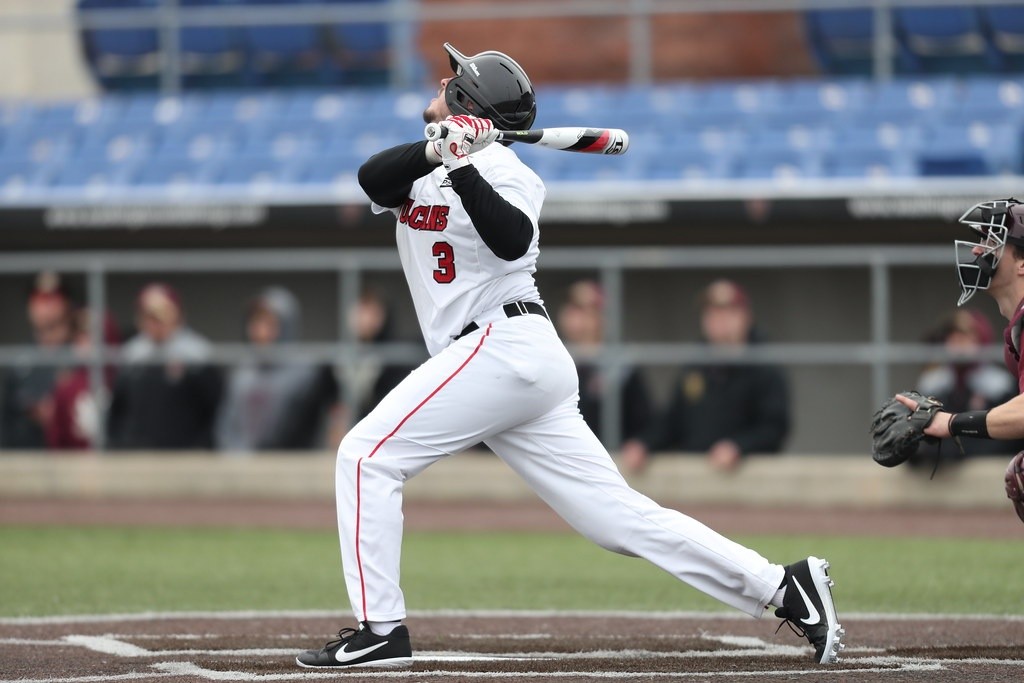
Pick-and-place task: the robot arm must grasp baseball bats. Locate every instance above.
[424,117,631,157]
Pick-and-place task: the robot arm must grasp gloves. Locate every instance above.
[433,116,499,164]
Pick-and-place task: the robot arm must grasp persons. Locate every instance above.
[874,198,1024,520]
[16,268,795,472]
[916,310,1020,411]
[296,41,846,669]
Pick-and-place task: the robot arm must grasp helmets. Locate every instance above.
[443,41,537,147]
[954,197,1024,307]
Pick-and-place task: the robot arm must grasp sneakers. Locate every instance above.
[774,555,846,666]
[296,623,413,668]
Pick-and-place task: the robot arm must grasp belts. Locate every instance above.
[451,303,549,340]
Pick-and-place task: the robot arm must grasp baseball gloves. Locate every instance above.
[867,389,968,480]
